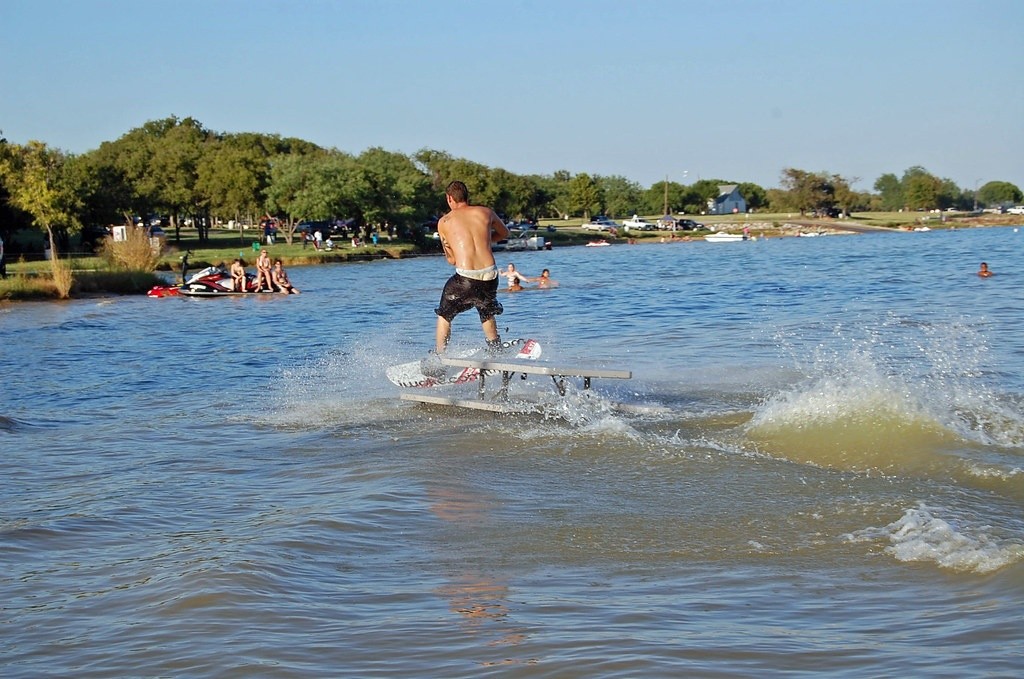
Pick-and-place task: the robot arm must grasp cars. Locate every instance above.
[581,219,621,231]
[590,216,608,221]
[505,218,540,231]
[671,218,704,232]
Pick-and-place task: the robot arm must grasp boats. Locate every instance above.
[704,230,751,242]
[178,268,296,299]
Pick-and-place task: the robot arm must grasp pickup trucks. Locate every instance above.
[623,214,655,231]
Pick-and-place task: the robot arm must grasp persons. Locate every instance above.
[240,204,1024,253]
[272,259,300,294]
[977,262,992,278]
[498,262,529,287]
[526,268,560,288]
[507,277,524,292]
[254,249,274,293]
[230,259,248,293]
[433,180,510,360]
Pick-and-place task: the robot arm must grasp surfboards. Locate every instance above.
[386,338,542,389]
